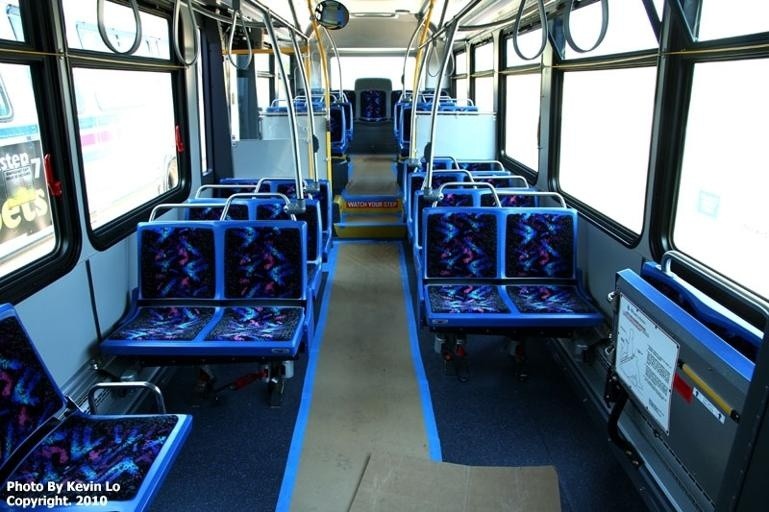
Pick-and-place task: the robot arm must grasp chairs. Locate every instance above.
[89,88,357,413]
[390,88,610,384]
[0,301,195,512]
[355,89,392,129]
